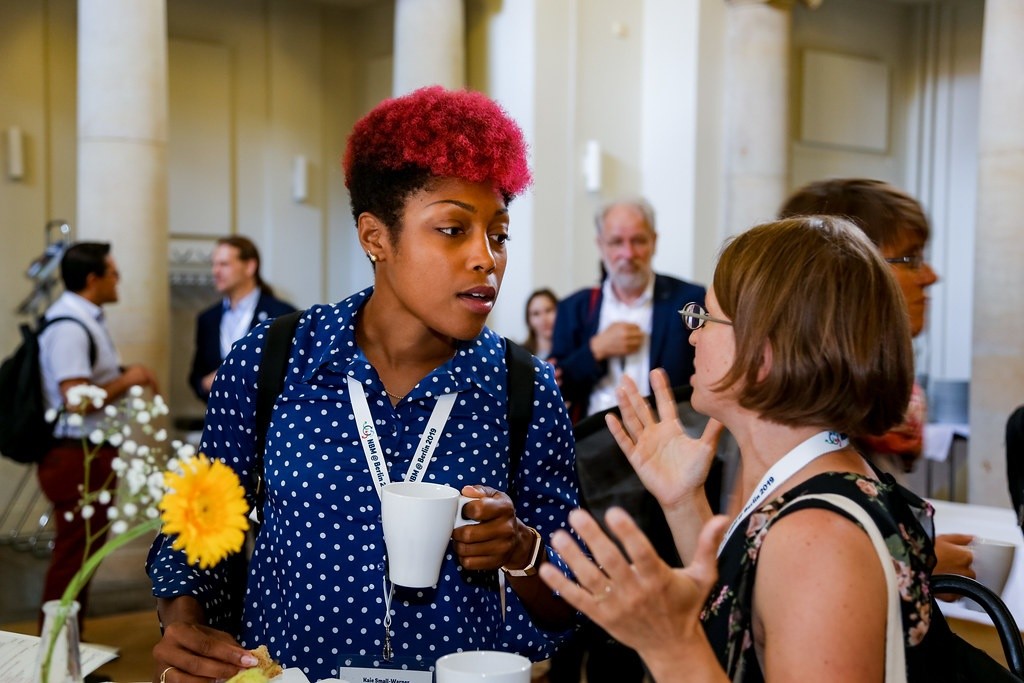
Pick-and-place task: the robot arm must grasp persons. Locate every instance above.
[39,243,159,640]
[521,289,558,363]
[538,216,939,683]
[550,197,710,424]
[145,86,584,683]
[776,178,976,602]
[188,237,295,402]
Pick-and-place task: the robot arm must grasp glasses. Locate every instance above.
[678,302,734,329]
[887,254,922,270]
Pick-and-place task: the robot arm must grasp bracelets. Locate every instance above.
[501,526,545,577]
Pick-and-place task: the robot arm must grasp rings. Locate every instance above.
[160,666,175,683]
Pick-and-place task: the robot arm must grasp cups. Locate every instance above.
[380,482,482,587]
[963,537,1015,613]
[435,650,532,682]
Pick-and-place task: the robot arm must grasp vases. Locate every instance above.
[29,601,81,683]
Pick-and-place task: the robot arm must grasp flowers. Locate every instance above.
[39,383,249,683]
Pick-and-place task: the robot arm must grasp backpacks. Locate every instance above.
[0,315,97,462]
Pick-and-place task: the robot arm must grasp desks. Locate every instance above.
[921,418,972,503]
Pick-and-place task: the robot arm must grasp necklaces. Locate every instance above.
[385,389,404,400]
